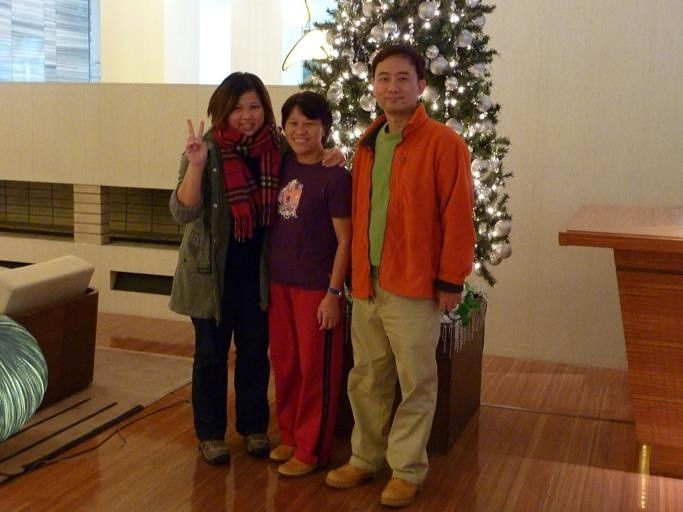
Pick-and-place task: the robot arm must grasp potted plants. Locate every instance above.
[269,1,511,460]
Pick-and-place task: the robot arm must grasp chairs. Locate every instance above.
[0,255,99,412]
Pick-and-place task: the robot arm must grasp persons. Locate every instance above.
[166,71,346,466]
[262,88,350,479]
[322,42,478,511]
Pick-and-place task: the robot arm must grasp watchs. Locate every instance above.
[325,287,343,298]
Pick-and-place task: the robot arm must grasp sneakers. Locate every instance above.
[199,439,230,464]
[270,445,296,460]
[278,458,317,476]
[247,433,270,457]
[380,478,421,507]
[325,462,386,489]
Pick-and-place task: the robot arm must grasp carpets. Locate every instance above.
[1,345,193,491]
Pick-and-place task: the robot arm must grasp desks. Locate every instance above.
[560,203,683,447]
